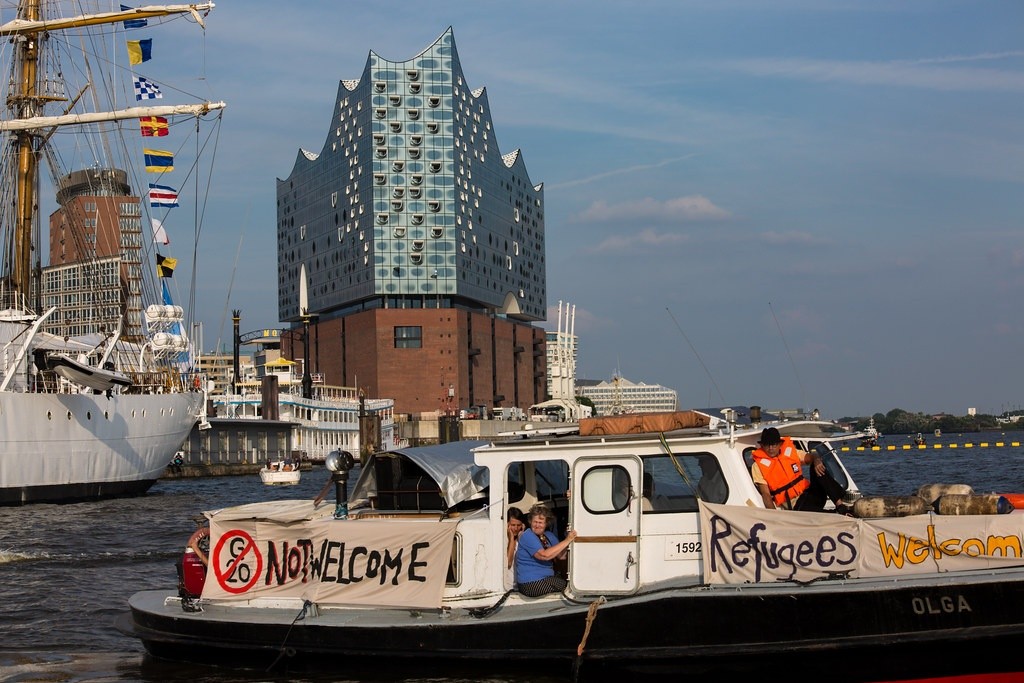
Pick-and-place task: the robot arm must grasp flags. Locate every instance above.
[133,76,162,101]
[140,115,169,136]
[151,220,169,246]
[156,254,177,279]
[127,38,153,67]
[144,148,174,174]
[120,6,148,30]
[149,183,179,208]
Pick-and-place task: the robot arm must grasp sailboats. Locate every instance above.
[0,0,226,506]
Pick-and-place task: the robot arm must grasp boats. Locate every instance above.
[258,461,302,488]
[127,408,1023,683]
[915,432,926,445]
[861,436,877,448]
[934,428,941,438]
[859,417,878,439]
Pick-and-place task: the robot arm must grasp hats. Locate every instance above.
[757,427,784,444]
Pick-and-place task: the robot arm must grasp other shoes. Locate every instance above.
[835,500,857,512]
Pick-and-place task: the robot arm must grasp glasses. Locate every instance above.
[763,441,779,448]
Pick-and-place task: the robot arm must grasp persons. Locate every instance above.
[693,453,727,505]
[813,409,820,421]
[751,427,860,518]
[515,505,576,597]
[643,471,654,512]
[507,506,525,590]
[174,452,184,465]
